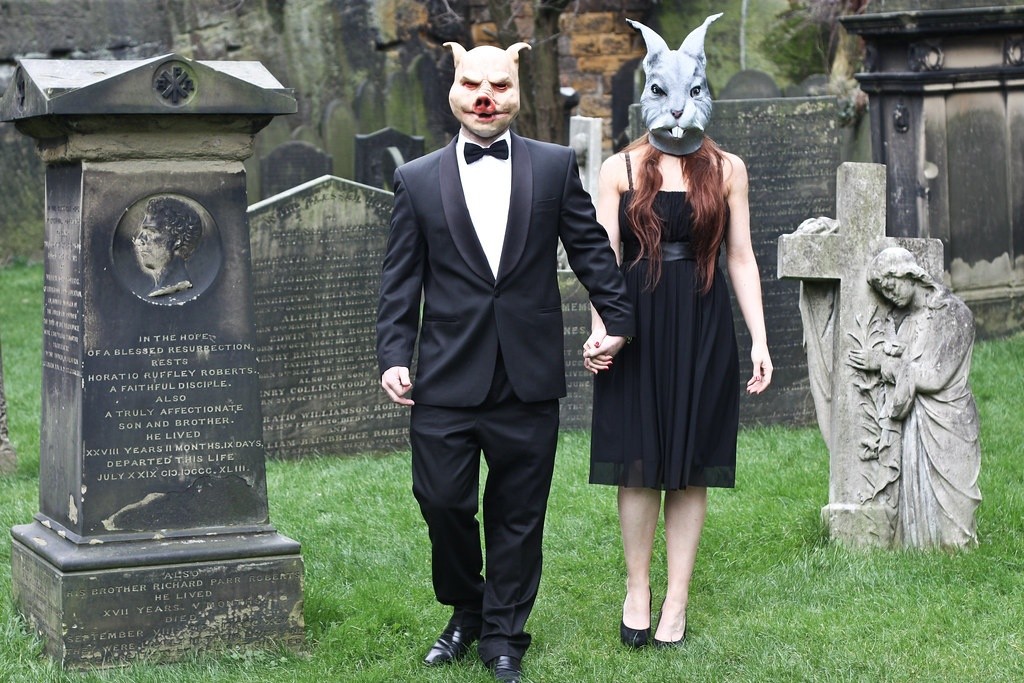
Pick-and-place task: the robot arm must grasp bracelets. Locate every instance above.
[625,336,632,344]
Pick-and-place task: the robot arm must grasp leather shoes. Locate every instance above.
[424,622,482,668]
[493,655,520,683]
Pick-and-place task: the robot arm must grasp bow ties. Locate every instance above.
[464,139,509,165]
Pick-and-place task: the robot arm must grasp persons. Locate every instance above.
[376,40,636,683]
[849,247,983,553]
[582,12,773,653]
[135,197,202,297]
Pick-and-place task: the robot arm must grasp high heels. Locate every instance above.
[653,596,687,653]
[621,585,652,649]
